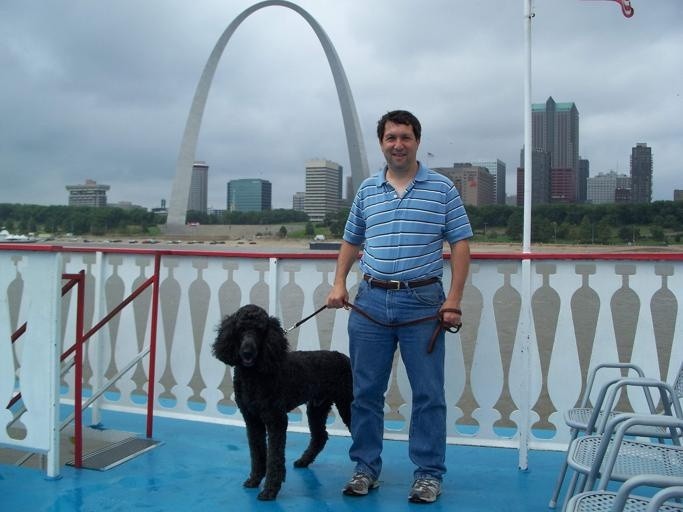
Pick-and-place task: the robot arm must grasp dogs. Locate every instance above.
[210,303,354,500]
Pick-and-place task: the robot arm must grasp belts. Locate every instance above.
[358,270,444,291]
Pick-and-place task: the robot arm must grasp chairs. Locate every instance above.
[549,358,683,512]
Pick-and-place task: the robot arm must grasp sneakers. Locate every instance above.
[407,476,443,506]
[339,472,382,497]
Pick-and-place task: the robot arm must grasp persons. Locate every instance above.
[323,108,474,504]
